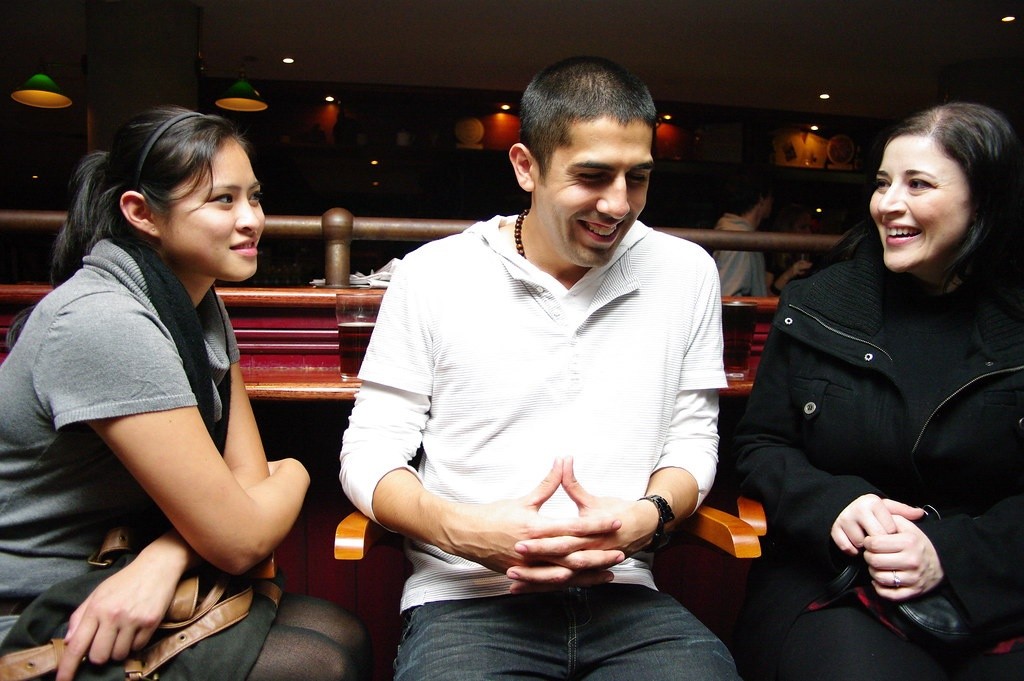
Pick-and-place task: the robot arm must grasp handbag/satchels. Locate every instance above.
[801,497,1024,655]
[0,525,284,681]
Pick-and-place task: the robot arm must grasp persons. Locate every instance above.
[0,104,370,681]
[731,102,1024,681]
[712,178,812,303]
[339,56,741,681]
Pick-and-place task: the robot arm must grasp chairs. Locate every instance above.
[335,512,760,563]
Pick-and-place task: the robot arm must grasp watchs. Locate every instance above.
[636,496,678,553]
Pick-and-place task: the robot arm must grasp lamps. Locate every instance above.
[200,61,270,113]
[10,53,86,109]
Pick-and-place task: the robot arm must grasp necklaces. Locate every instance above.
[514,207,534,262]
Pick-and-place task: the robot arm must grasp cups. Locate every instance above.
[720,300,759,381]
[334,295,382,379]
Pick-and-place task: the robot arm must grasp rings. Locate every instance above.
[889,571,909,593]
